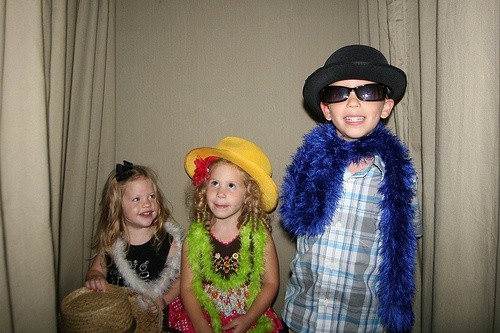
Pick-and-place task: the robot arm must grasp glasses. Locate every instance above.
[319,84,388,103]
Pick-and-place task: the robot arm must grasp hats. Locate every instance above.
[184,136,278,214]
[60,283,161,333]
[303,45,407,124]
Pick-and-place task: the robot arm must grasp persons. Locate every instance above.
[167,136,284,333]
[82,160,182,333]
[277,45,419,333]
